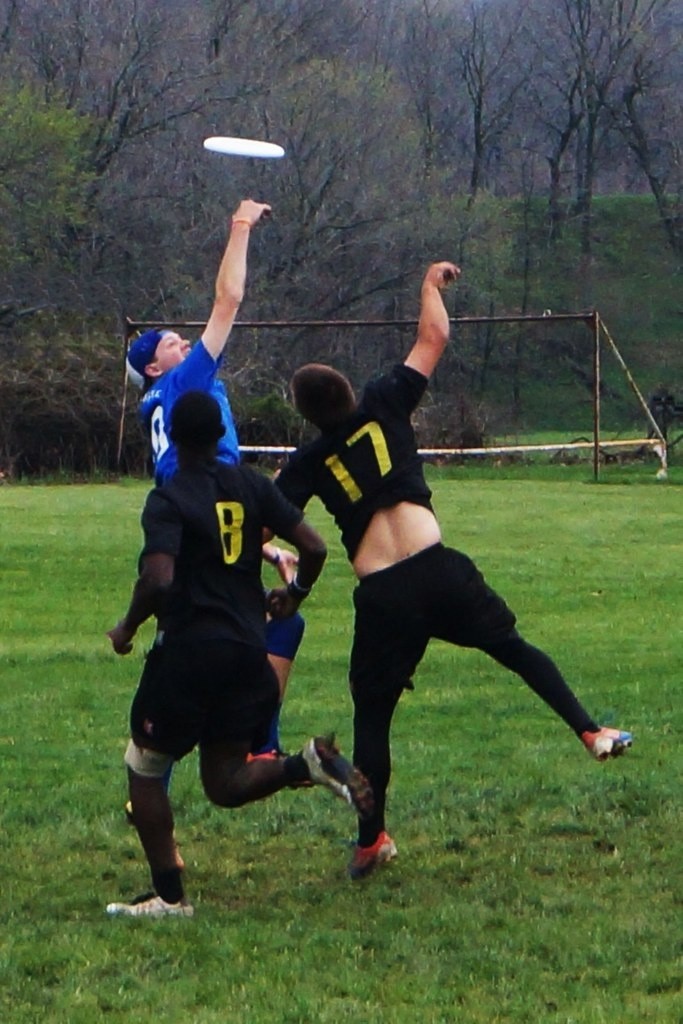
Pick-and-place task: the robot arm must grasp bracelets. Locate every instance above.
[232,218,253,231]
[270,546,280,566]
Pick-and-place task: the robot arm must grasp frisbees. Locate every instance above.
[203,136,286,159]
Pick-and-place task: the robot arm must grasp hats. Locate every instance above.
[129,327,170,395]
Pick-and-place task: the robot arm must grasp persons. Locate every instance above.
[127,198,306,816]
[263,261,634,877]
[107,392,376,919]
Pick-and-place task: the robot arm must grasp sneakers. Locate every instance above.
[302,737,375,820]
[346,832,399,879]
[584,724,633,760]
[106,896,197,915]
